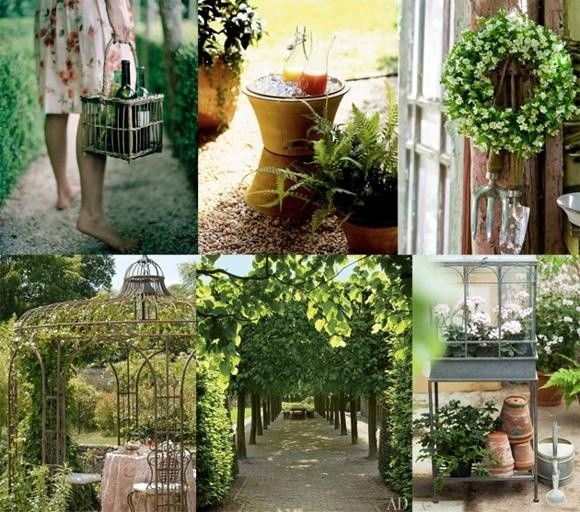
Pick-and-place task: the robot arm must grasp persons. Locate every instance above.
[33,1,143,254]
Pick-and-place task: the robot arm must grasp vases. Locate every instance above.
[531,370,566,406]
[482,395,535,477]
[240,71,352,157]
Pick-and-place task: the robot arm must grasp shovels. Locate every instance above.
[499,73,532,255]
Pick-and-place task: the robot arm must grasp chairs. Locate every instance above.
[126,441,193,512]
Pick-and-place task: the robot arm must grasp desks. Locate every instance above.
[106,449,191,512]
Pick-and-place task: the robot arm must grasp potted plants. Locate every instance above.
[411,398,503,493]
[239,77,400,254]
[199,0,272,133]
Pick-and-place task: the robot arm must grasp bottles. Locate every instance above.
[284,22,336,96]
[106,60,150,151]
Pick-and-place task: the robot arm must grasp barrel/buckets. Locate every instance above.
[538,421,575,487]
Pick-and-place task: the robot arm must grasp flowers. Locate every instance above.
[431,255,580,372]
[438,8,580,162]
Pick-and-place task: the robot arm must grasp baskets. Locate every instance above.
[81,37,166,166]
